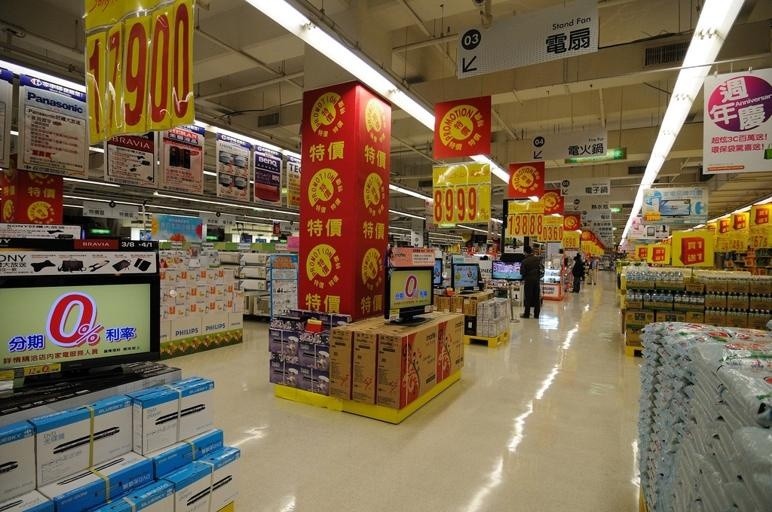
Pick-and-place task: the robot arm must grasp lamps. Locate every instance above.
[618,0,745,250]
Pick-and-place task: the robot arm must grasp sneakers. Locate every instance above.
[520,312,541,319]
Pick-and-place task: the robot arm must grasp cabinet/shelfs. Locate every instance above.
[540,256,566,301]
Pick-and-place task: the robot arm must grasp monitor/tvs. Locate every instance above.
[451,263,480,293]
[434,258,442,288]
[492,260,524,281]
[385,267,433,324]
[0,238,160,393]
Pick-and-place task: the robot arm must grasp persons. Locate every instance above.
[519,246,543,319]
[587,256,598,286]
[569,256,584,294]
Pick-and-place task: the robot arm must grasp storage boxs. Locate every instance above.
[158,248,246,320]
[3,375,242,512]
[328,311,465,409]
[435,288,490,336]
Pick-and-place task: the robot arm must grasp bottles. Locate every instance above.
[619,269,771,318]
